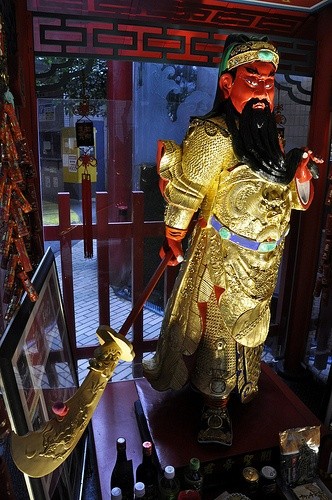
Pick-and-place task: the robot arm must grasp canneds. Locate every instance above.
[241,467,261,493]
[260,466,277,494]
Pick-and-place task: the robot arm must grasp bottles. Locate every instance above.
[136,441,156,491]
[110,438,134,500]
[134,482,148,500]
[159,465,180,500]
[184,458,203,493]
[111,487,122,500]
